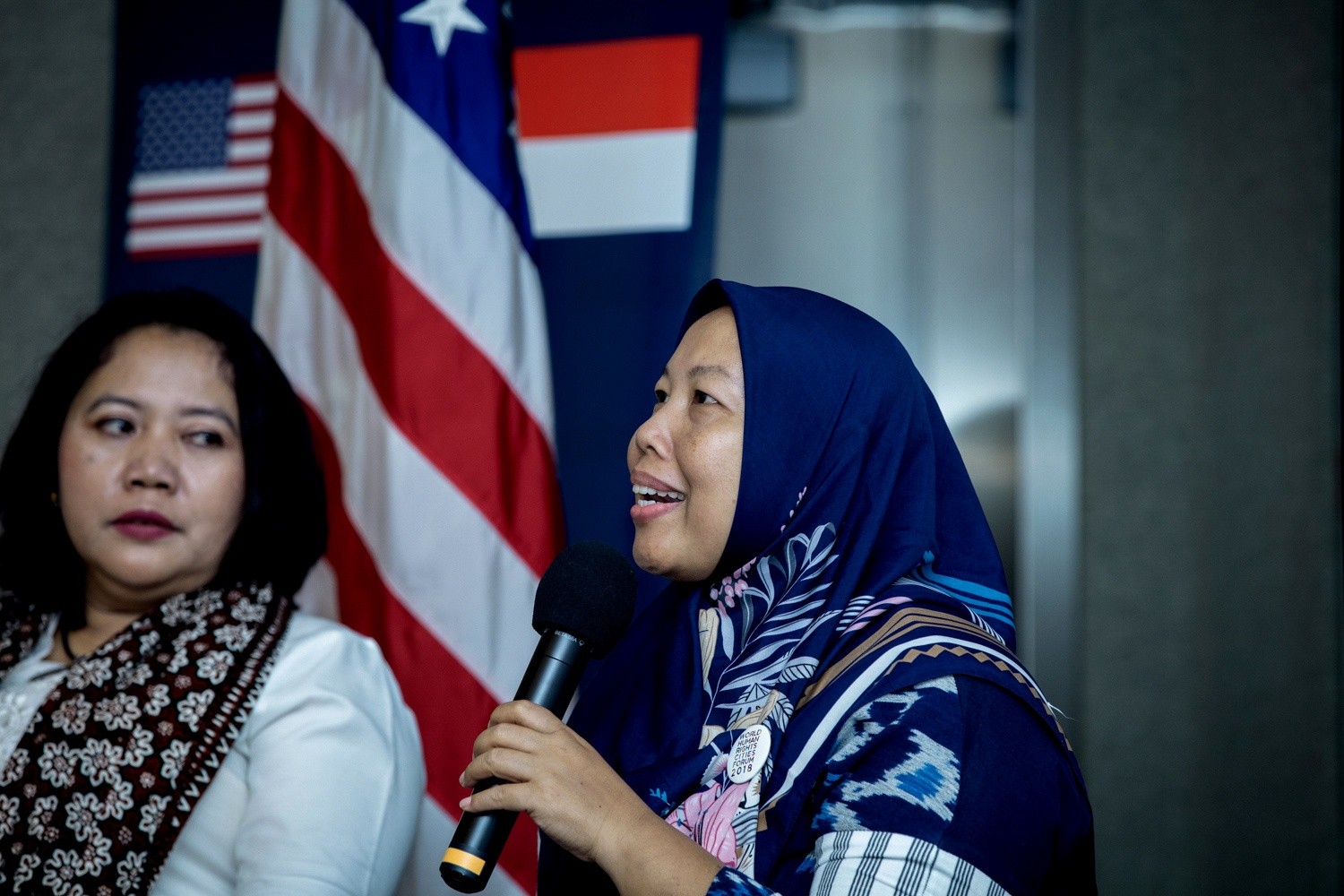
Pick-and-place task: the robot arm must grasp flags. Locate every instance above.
[251,1,564,896]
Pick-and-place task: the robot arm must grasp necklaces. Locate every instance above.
[58,630,81,661]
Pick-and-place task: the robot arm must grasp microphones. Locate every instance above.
[439,539,638,893]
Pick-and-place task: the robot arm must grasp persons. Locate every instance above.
[457,276,1096,896]
[0,288,429,896]
